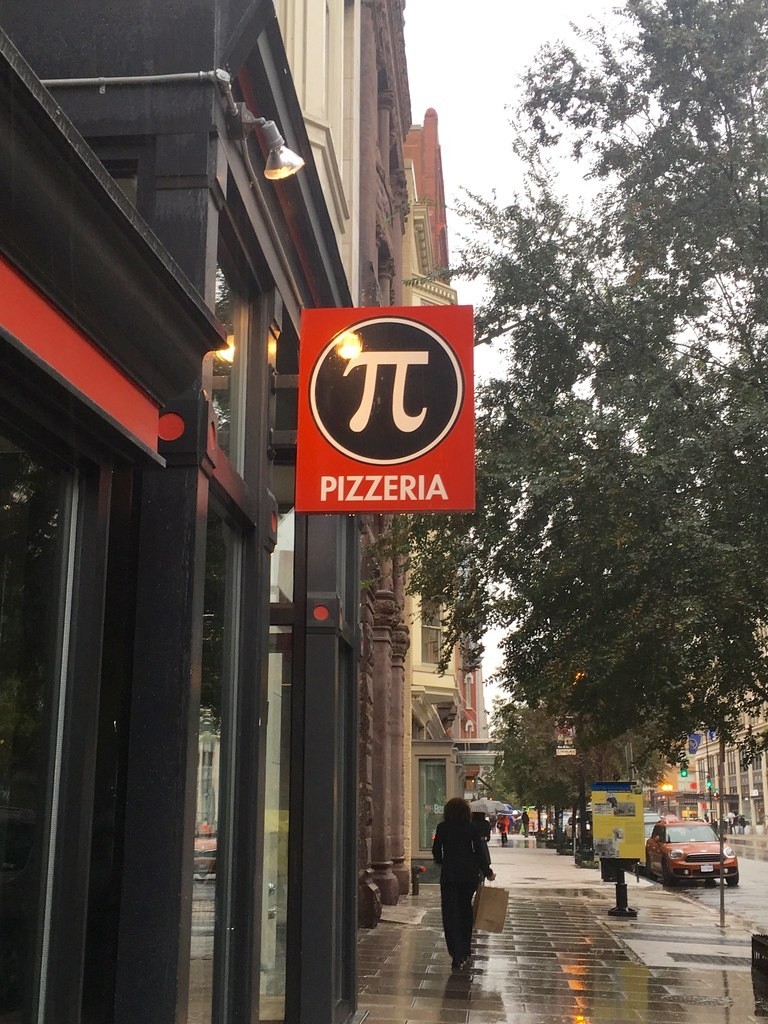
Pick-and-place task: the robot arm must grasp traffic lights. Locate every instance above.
[707,776,711,787]
[680,763,689,777]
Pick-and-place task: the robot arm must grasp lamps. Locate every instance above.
[226,101,306,181]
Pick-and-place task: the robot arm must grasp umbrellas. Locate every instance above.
[466,799,522,815]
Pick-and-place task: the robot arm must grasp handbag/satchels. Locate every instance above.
[472,879,509,933]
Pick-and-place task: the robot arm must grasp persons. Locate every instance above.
[432,797,497,967]
[470,800,493,865]
[497,808,529,848]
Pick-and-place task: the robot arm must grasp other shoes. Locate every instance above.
[452,950,472,968]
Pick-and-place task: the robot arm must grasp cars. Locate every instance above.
[521,805,595,848]
[643,811,661,868]
[644,816,739,888]
